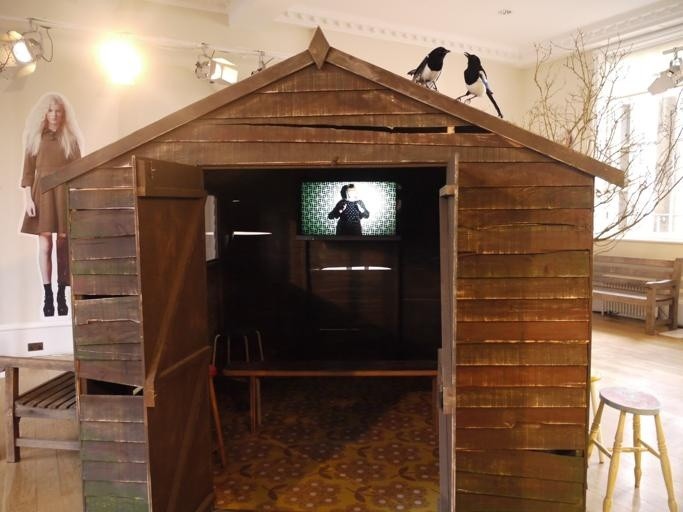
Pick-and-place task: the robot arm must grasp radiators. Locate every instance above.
[602,281,647,321]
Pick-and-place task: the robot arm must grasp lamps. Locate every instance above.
[193,44,239,86]
[1,18,53,78]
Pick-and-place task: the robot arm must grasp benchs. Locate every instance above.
[225,360,438,435]
[1,356,84,464]
[594,255,682,334]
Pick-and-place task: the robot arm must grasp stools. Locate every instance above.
[587,375,680,512]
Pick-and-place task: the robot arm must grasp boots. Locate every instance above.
[43,283,54,317]
[56,284,68,316]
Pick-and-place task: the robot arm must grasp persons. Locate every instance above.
[328,184,369,235]
[20,92,81,317]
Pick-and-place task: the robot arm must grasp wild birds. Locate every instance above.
[407,46,451,92]
[455,52,503,118]
